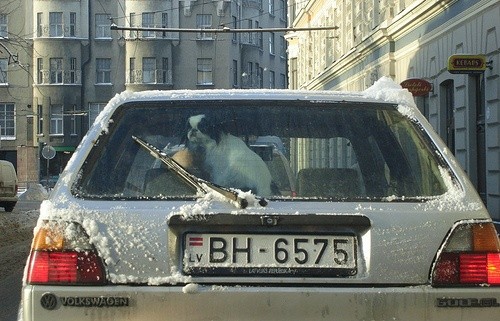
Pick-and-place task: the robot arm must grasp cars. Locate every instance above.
[18,88,499,321]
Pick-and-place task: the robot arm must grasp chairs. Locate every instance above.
[144,166,199,196]
[296,166,362,198]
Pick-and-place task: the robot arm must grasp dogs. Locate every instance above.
[178,114,282,197]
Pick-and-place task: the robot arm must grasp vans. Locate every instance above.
[0,160,18,212]
